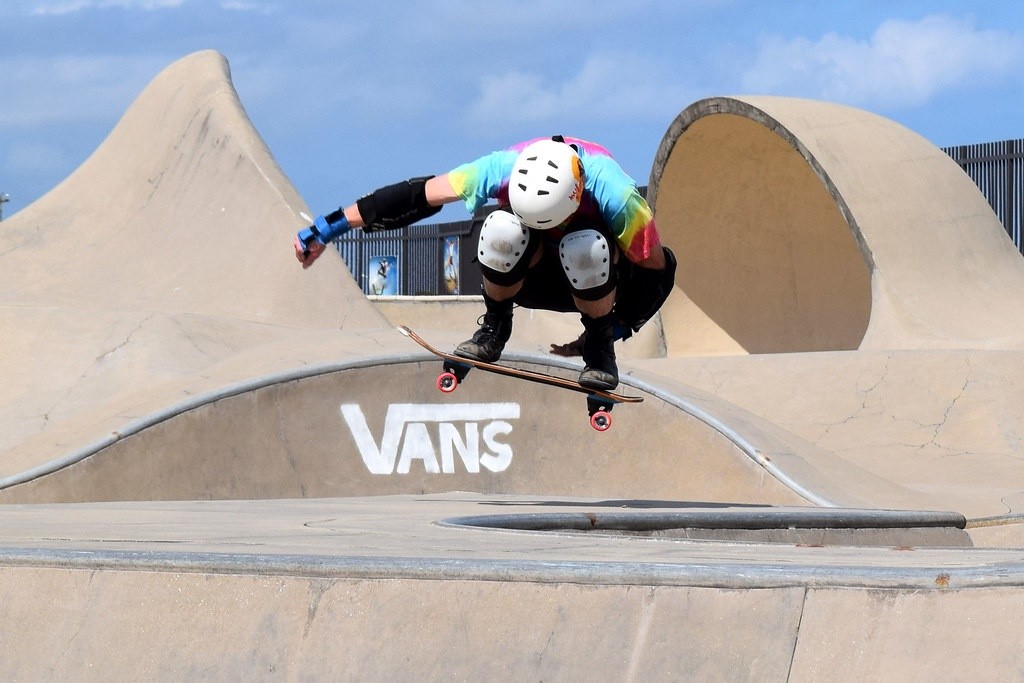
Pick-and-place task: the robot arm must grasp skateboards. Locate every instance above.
[398,321,644,431]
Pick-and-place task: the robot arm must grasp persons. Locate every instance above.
[444,237,458,284]
[294,135,677,389]
[378,258,388,274]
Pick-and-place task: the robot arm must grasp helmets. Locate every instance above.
[507,134,586,232]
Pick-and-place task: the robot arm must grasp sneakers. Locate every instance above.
[579,348,618,390]
[454,319,513,363]
